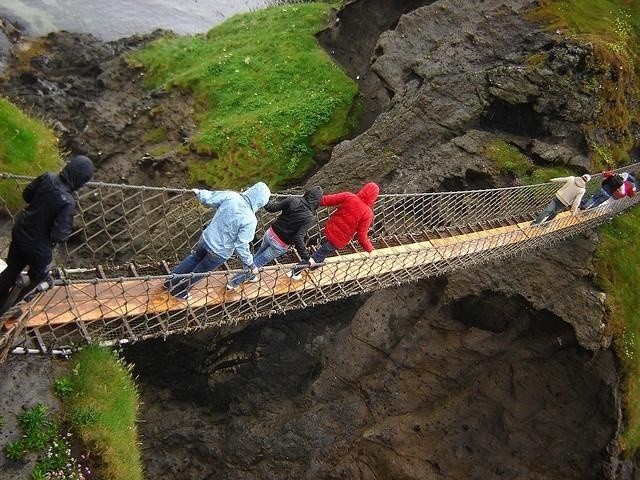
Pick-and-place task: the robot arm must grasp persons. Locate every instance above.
[162,182,271,296]
[580,171,628,211]
[286,182,380,281]
[529,174,591,229]
[0,156,94,317]
[226,186,324,290]
[600,171,640,208]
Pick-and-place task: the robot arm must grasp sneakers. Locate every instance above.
[285,270,302,279]
[244,275,260,283]
[225,284,244,292]
[162,283,192,301]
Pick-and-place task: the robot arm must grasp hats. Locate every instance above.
[582,174,591,182]
